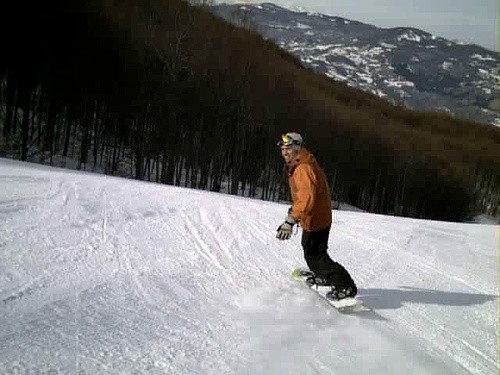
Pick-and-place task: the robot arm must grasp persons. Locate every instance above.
[276,132,357,301]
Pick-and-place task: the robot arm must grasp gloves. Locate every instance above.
[276,217,295,240]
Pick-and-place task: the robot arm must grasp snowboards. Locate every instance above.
[292,267,372,314]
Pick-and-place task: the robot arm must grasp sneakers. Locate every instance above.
[325,281,357,300]
[306,274,334,286]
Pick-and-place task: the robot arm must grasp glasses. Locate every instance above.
[276,135,303,146]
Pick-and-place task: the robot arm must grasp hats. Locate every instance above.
[286,133,304,149]
